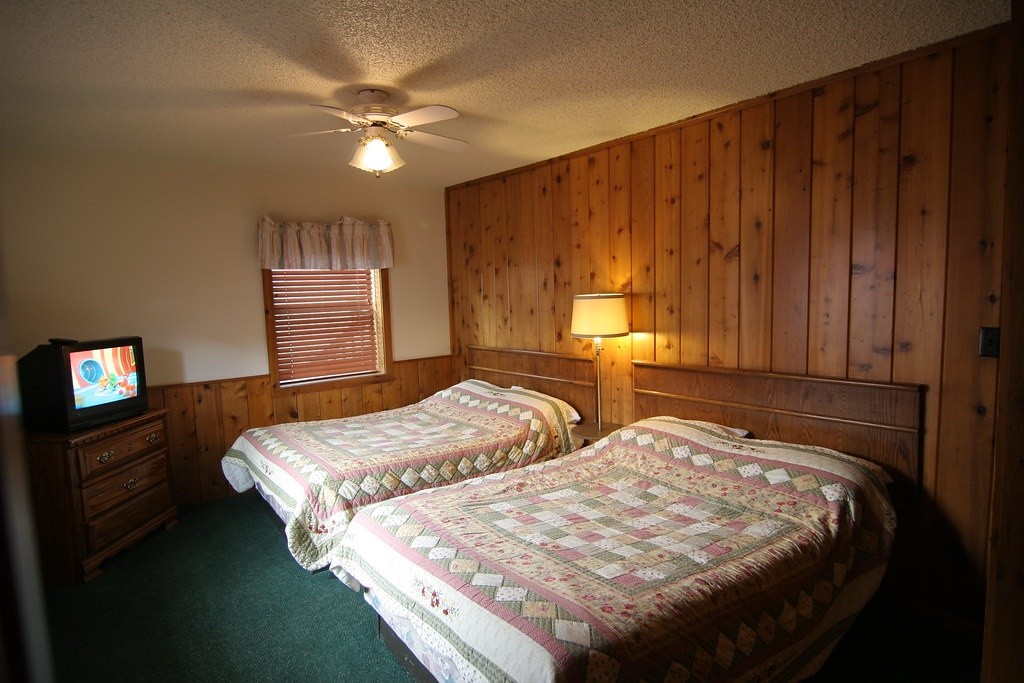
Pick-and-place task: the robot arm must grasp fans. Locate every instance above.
[256,89,468,153]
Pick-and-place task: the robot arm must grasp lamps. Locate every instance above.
[347,135,407,179]
[570,292,630,432]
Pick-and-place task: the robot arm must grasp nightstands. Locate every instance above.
[570,422,626,447]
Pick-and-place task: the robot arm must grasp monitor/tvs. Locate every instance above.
[17,335,149,437]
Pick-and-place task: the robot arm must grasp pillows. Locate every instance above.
[609,416,880,520]
[444,378,581,430]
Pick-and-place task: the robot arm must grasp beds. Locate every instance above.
[220,346,598,573]
[328,359,929,683]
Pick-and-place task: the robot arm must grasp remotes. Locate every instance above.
[47,338,78,345]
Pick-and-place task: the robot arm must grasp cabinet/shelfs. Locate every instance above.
[22,407,181,585]
